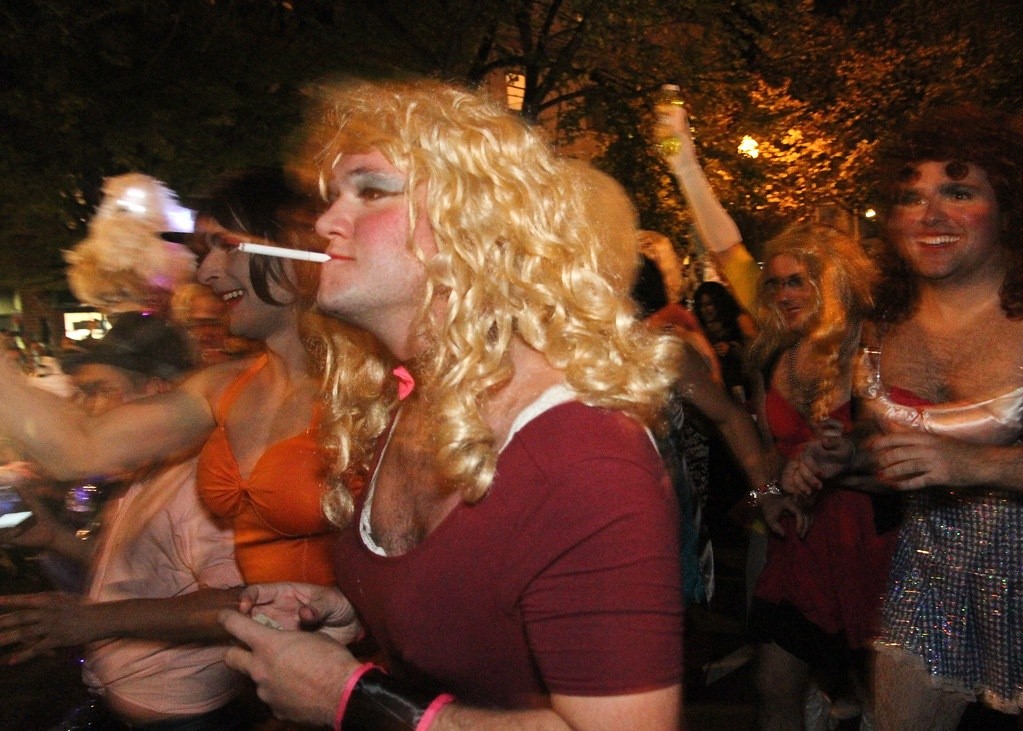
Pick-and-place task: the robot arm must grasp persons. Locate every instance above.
[0,78,1023,730]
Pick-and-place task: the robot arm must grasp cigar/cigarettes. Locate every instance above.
[238,243,331,263]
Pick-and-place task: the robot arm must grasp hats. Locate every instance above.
[59,310,194,385]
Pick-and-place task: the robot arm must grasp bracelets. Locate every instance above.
[747,481,781,508]
[334,663,454,731]
